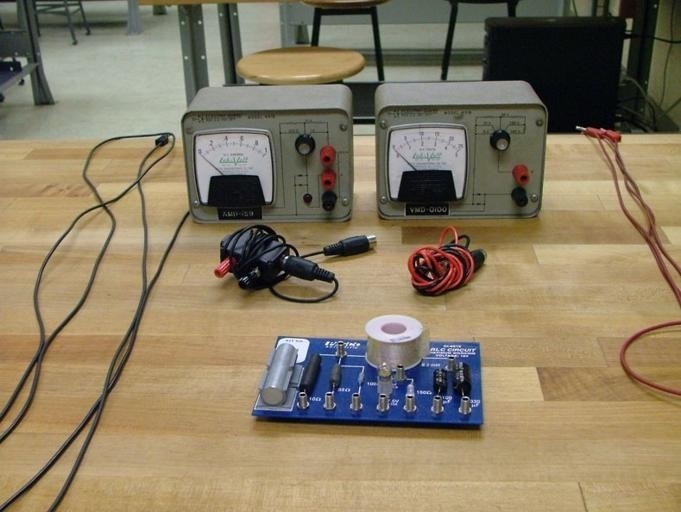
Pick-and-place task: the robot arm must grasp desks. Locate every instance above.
[0,134,681,512]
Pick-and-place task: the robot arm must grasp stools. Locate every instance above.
[440,1,521,79]
[311,4,390,81]
[235,46,367,85]
